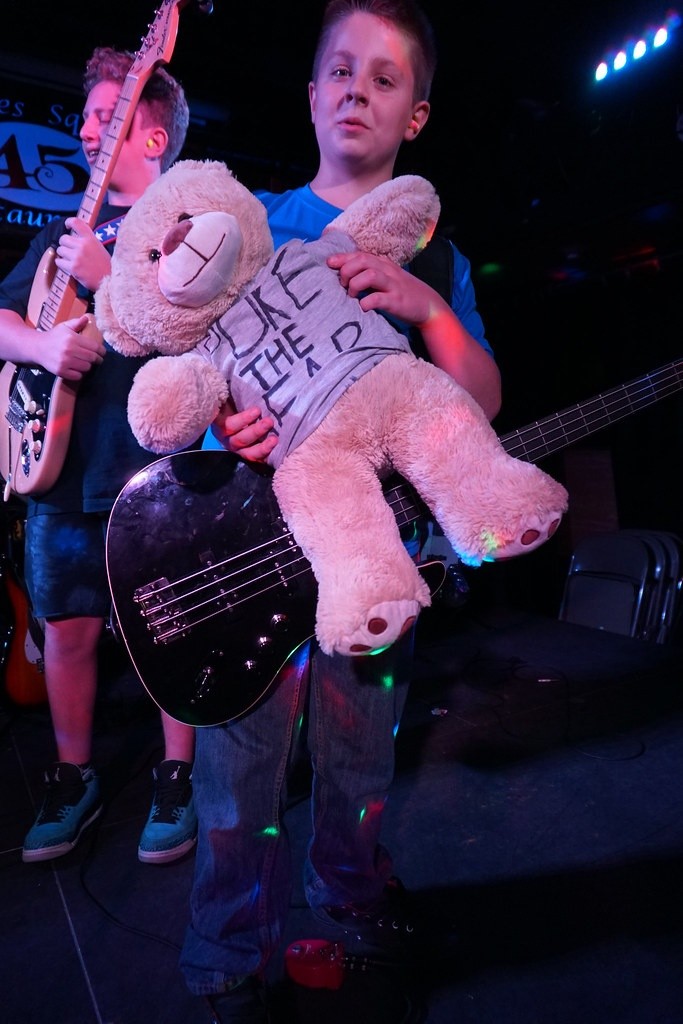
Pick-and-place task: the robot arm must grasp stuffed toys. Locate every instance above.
[92,158,569,661]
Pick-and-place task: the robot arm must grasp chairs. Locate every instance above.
[558,528,683,645]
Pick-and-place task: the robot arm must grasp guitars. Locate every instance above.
[97,358,683,729]
[0,1,185,500]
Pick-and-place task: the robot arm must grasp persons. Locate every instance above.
[0,50,220,863]
[183,11,503,1024]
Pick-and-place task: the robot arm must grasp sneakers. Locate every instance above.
[134,759,197,864]
[199,972,277,1024]
[307,876,475,980]
[21,758,106,863]
